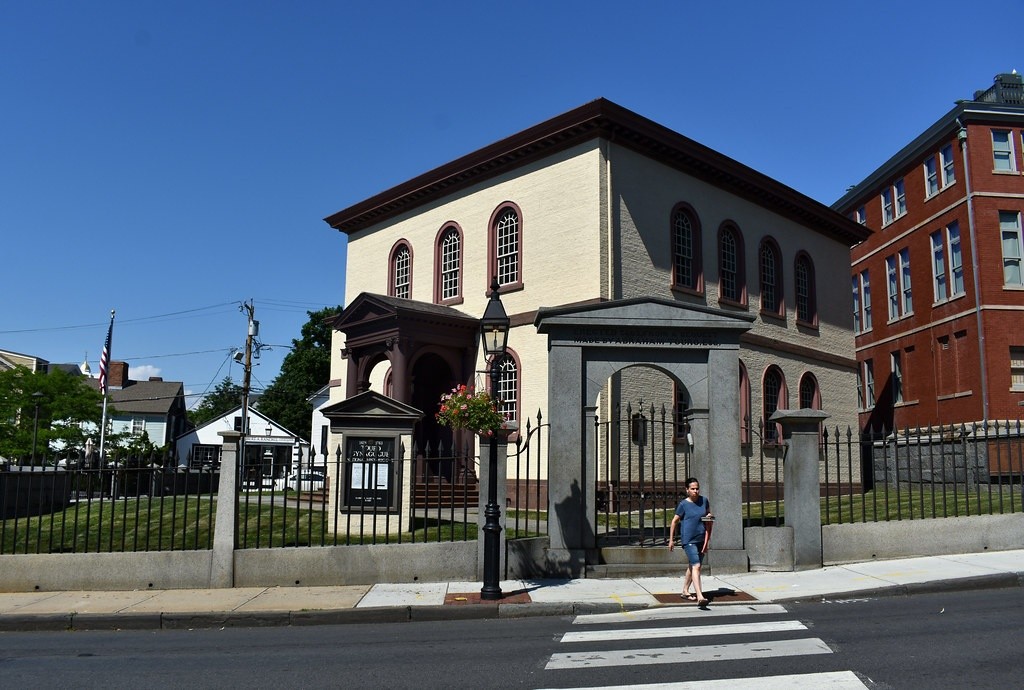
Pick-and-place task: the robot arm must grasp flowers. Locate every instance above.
[435,384,511,437]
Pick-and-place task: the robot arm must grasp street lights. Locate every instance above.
[479,276,511,600]
[30,388,43,471]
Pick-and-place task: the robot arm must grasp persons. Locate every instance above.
[669,478,713,606]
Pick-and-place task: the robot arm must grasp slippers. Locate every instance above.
[680,594,695,601]
[698,599,710,606]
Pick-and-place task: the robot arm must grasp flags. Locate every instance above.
[100,324,112,396]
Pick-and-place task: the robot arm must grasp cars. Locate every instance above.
[274,470,325,492]
